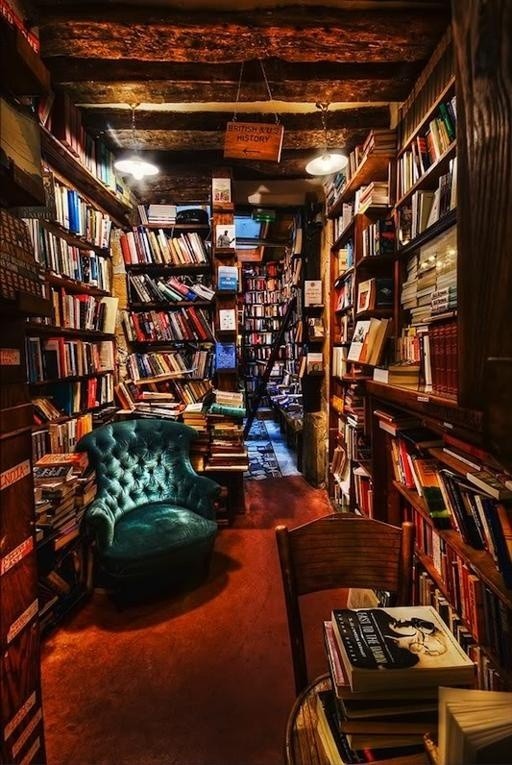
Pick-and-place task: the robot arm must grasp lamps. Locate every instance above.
[113,154,159,182]
[304,151,350,177]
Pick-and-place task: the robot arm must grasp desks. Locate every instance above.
[285,660,375,765]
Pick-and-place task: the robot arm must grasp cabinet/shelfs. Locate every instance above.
[319,149,398,522]
[130,220,215,399]
[365,119,509,432]
[21,114,134,632]
[366,398,512,643]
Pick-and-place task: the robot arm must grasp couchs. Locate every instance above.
[77,416,222,611]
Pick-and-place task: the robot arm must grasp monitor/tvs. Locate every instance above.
[234,216,262,250]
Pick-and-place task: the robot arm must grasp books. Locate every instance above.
[114,379,213,472]
[123,306,215,343]
[127,350,207,380]
[333,202,353,243]
[395,324,459,401]
[304,280,323,308]
[374,403,512,601]
[332,311,352,378]
[241,249,304,425]
[396,495,512,678]
[136,202,178,224]
[323,127,397,211]
[220,309,236,331]
[21,218,115,293]
[19,333,120,384]
[391,95,456,196]
[315,605,475,764]
[34,281,119,335]
[307,353,323,375]
[418,564,510,692]
[216,342,235,369]
[127,271,215,303]
[218,266,237,290]
[210,390,250,471]
[435,686,512,765]
[362,219,396,256]
[347,319,389,367]
[329,385,371,516]
[36,82,131,207]
[397,158,458,243]
[42,170,116,252]
[32,414,101,542]
[398,223,459,320]
[356,277,377,315]
[119,225,209,265]
[31,373,116,423]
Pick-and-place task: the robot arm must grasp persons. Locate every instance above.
[218,226,234,247]
[309,361,322,376]
[331,605,449,672]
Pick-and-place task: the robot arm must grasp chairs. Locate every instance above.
[275,509,419,703]
